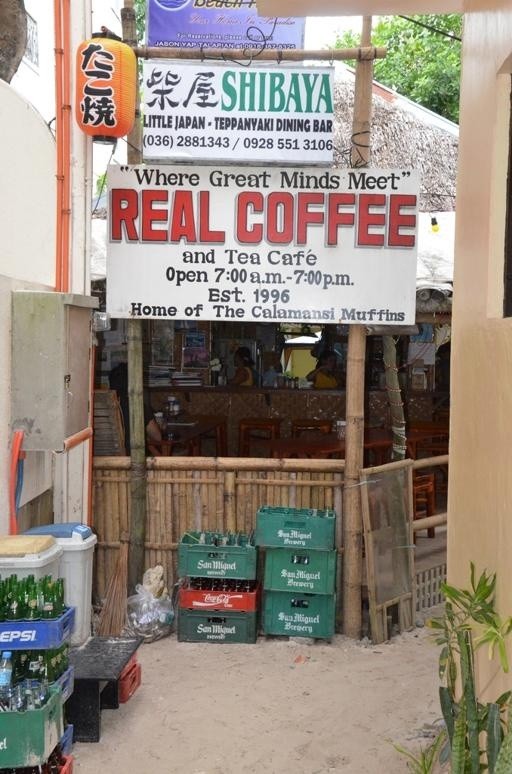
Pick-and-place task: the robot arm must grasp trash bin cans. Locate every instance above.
[0,523,97,647]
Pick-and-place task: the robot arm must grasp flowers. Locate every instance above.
[209,357,222,371]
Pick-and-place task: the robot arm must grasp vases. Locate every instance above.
[211,370,218,385]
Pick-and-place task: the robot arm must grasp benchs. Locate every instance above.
[64,633,145,742]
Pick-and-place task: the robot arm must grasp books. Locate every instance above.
[144,362,203,387]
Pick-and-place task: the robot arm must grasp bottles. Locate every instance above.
[192,530,257,594]
[323,506,331,517]
[0,575,70,710]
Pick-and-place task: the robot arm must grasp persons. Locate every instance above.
[368,358,382,386]
[108,363,162,457]
[307,351,338,388]
[230,346,255,386]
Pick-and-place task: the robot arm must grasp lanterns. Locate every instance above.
[73,31,138,146]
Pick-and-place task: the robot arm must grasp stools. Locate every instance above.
[237,417,332,457]
[384,459,435,543]
